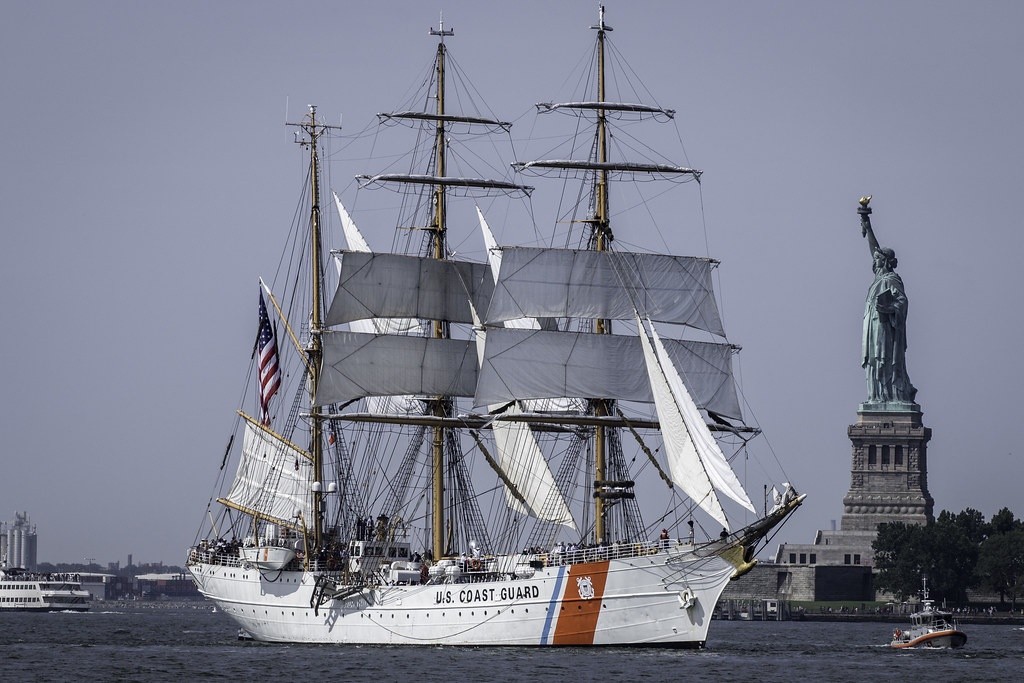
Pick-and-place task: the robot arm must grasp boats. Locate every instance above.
[890,575,968,650]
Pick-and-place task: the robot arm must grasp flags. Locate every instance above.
[257,285,282,428]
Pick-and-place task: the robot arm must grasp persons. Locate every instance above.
[860,215,918,403]
[792,599,1019,618]
[198,512,731,587]
[895,627,902,641]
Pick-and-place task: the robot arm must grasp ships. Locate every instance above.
[0,511,92,611]
[187,0,807,652]
